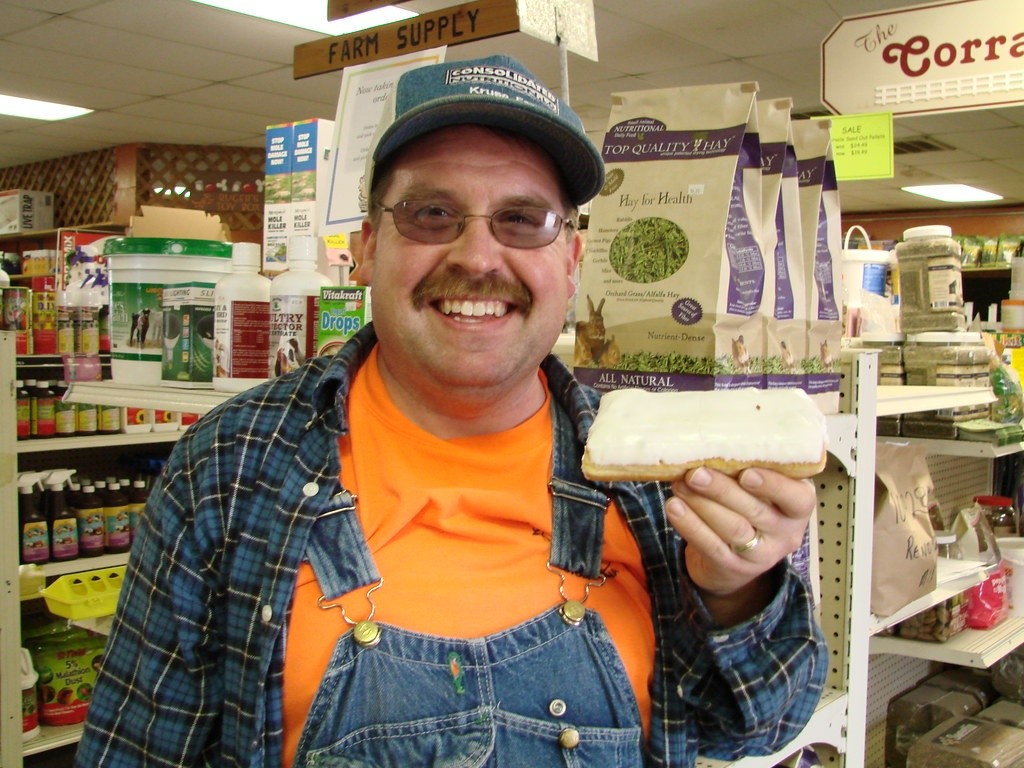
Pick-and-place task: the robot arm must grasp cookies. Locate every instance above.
[581,388,827,483]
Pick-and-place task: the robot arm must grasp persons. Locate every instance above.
[72,54,829,768]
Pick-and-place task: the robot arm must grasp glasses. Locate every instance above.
[373,197,574,249]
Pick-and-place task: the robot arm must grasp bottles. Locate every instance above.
[974,494,1020,539]
[20,641,41,744]
[899,529,969,643]
[15,379,173,566]
[212,241,273,393]
[903,331,991,440]
[268,235,333,380]
[860,331,905,437]
[895,226,966,334]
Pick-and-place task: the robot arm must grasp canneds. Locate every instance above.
[0,275,110,358]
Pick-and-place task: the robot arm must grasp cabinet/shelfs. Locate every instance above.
[0,330,1024,768]
[0,222,129,279]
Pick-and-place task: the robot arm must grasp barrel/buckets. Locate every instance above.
[838,224,891,314]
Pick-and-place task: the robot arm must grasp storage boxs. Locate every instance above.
[258,117,335,280]
[0,190,54,234]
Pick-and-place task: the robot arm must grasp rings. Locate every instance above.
[729,527,761,552]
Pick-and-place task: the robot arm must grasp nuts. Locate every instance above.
[897,595,956,642]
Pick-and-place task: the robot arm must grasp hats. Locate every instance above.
[367,54,606,220]
